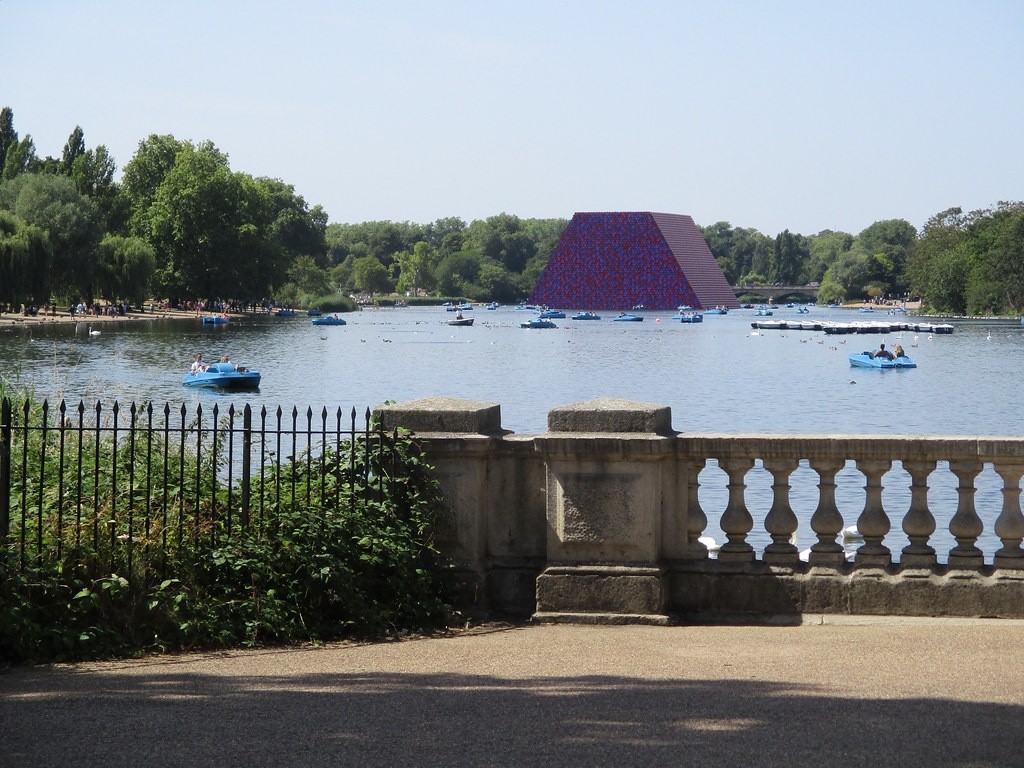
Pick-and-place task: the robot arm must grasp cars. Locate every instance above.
[805,281,819,286]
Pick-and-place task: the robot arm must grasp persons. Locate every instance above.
[874,344,889,358]
[221,353,239,371]
[0,292,297,320]
[191,353,208,373]
[450,300,727,325]
[895,345,904,358]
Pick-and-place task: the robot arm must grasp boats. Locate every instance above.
[274,311,297,316]
[741,300,909,317]
[677,305,692,311]
[447,318,474,325]
[307,312,321,316]
[202,316,229,324]
[704,308,727,314]
[751,319,955,337]
[312,316,346,326]
[672,314,703,323]
[180,362,261,392]
[613,315,644,322]
[442,301,549,311]
[571,313,601,320]
[847,349,917,370]
[538,310,566,318]
[633,306,642,311]
[521,320,556,329]
